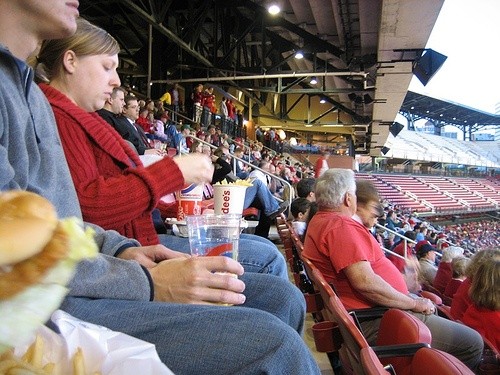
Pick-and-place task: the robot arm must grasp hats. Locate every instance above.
[438,234,446,238]
[159,113,171,120]
[442,243,449,247]
[415,240,438,256]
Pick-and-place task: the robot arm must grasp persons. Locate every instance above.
[302,168,484,375]
[0,0,322,375]
[31,17,500,356]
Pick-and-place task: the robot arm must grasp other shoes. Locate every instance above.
[268,206,287,220]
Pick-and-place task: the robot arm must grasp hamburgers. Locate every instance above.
[0,189,99,301]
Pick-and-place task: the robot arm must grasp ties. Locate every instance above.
[133,123,149,147]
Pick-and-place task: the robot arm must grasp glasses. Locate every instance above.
[128,105,141,109]
[367,203,381,212]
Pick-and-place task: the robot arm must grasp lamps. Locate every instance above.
[373,145,390,155]
[378,121,405,137]
[391,46,449,87]
[319,74,327,104]
[295,25,305,60]
[348,92,375,107]
[310,50,318,85]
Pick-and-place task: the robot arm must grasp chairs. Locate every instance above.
[276,213,500,375]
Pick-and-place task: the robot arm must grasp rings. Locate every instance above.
[430,308,434,311]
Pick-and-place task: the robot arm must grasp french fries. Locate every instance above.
[0,333,102,375]
[215,177,254,186]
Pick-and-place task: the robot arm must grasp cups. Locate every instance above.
[176,201,202,220]
[186,214,239,278]
[212,184,248,239]
[179,182,204,201]
